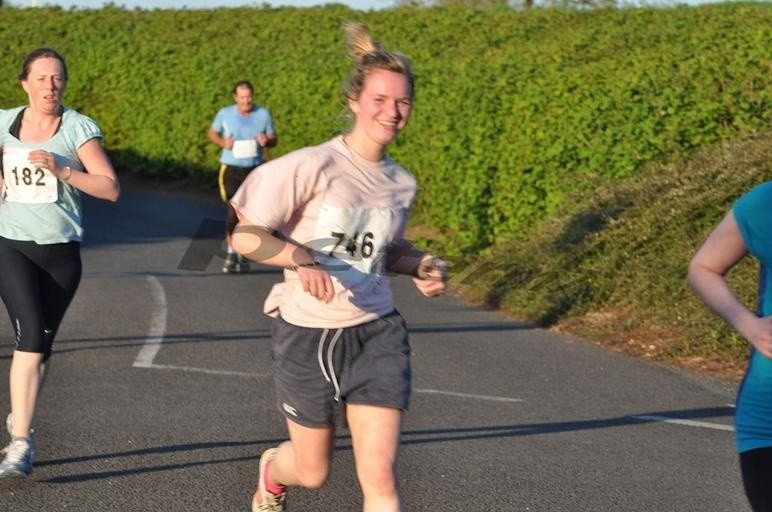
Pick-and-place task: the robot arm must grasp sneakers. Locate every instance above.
[0,413,35,479]
[222,254,249,273]
[252,448,287,512]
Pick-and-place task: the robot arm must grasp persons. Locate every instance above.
[684,183,772,512]
[208,81,280,275]
[1,48,121,478]
[229,23,452,511]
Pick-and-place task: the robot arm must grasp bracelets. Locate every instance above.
[59,166,71,181]
[292,261,320,273]
